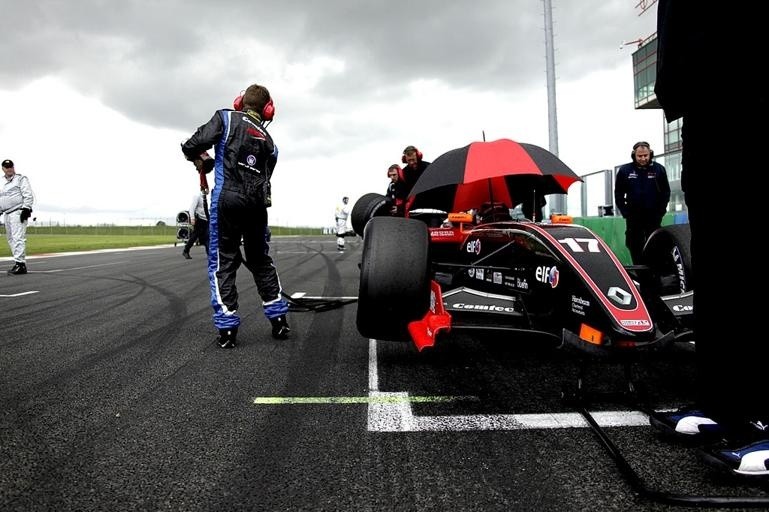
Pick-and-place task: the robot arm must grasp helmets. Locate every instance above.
[479,201,511,221]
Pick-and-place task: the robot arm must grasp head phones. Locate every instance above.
[233,90,275,121]
[631,143,654,159]
[402,148,422,163]
[387,163,405,181]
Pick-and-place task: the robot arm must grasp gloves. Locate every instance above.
[19,210,32,223]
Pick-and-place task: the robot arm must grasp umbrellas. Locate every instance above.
[408,130,587,224]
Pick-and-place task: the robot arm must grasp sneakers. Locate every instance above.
[648,402,728,446]
[7,263,28,274]
[270,315,290,339]
[214,328,238,348]
[182,251,193,260]
[698,419,769,481]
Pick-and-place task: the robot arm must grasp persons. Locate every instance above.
[0,159,33,275]
[182,85,289,350]
[334,197,349,251]
[182,186,210,259]
[395,146,431,204]
[386,164,404,216]
[615,141,671,267]
[522,195,546,222]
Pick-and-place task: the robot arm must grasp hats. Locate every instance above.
[2,159,14,167]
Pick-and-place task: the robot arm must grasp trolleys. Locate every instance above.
[174,210,200,247]
